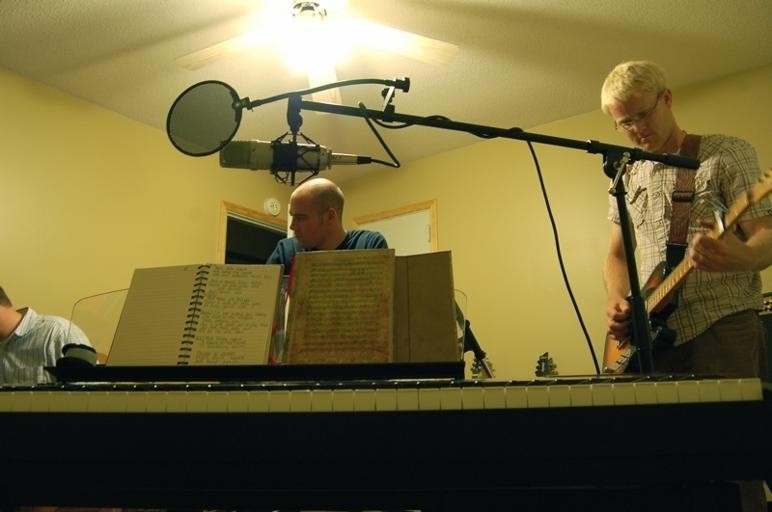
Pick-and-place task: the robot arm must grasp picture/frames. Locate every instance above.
[353,198,439,254]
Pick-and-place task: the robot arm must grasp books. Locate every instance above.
[279,248,398,363]
[105,264,284,369]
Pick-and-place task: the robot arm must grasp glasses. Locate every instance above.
[615,95,660,133]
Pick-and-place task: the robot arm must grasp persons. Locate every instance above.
[0,285,101,391]
[263,178,388,267]
[600,55,772,381]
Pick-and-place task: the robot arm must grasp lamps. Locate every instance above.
[288,3,328,28]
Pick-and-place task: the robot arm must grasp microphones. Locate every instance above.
[220,138,371,172]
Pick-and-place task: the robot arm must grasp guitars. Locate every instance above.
[602,169,772,376]
